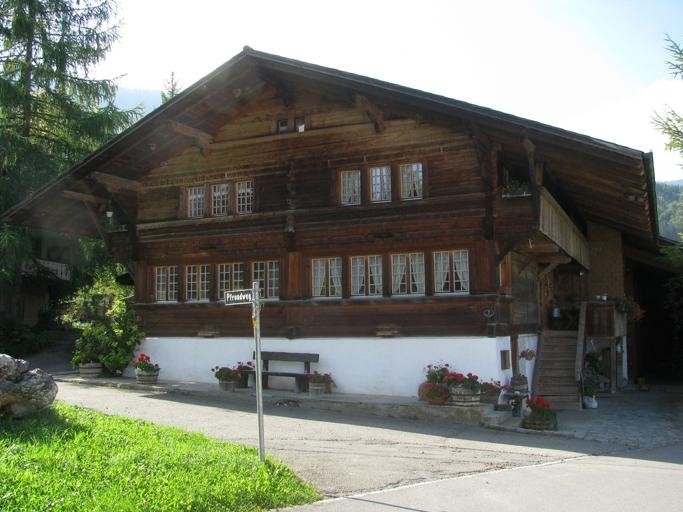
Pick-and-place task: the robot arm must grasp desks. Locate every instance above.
[554,305,580,329]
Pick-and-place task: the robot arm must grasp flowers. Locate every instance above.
[130,353,156,372]
[419,363,553,418]
[211,361,255,382]
[81,350,97,364]
[307,371,336,388]
[613,295,647,320]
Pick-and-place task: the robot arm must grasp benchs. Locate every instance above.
[240,349,320,394]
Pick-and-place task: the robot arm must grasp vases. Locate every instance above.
[309,382,331,395]
[523,415,557,430]
[236,375,248,388]
[219,382,236,392]
[78,363,102,378]
[133,368,160,386]
